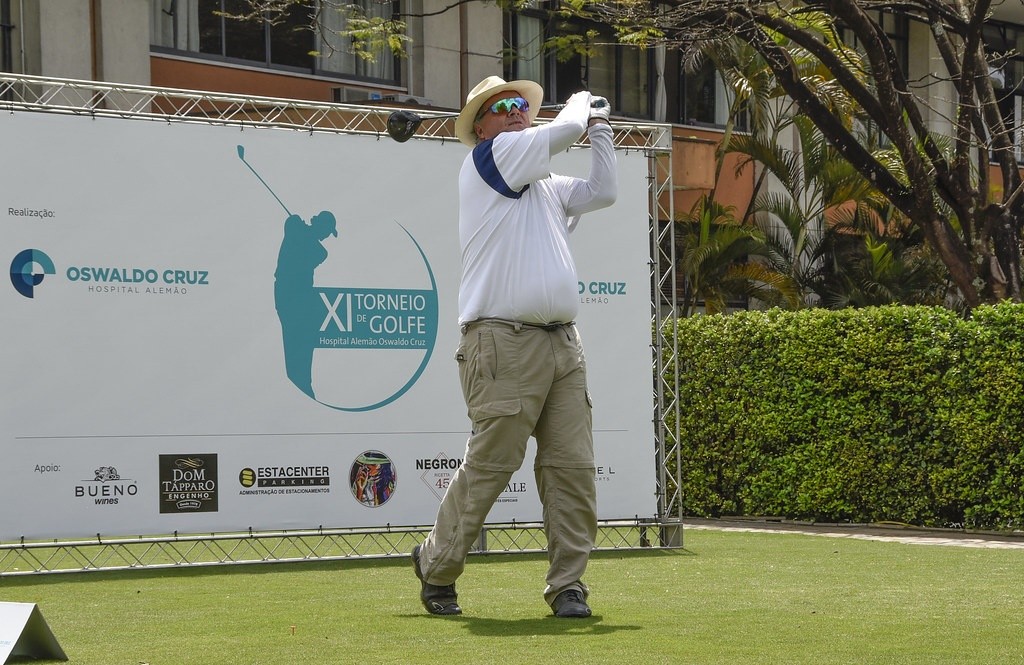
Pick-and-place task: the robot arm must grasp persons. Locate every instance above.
[411,76,620,617]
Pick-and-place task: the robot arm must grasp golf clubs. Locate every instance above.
[386,100,606,144]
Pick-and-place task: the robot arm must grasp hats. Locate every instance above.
[454,75,544,147]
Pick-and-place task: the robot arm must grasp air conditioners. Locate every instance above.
[384,94,432,107]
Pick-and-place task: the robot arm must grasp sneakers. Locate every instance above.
[411,545,462,614]
[551,589,592,617]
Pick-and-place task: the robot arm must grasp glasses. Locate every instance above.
[479,97,529,120]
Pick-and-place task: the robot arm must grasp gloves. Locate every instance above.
[588,96,611,126]
[572,91,591,102]
